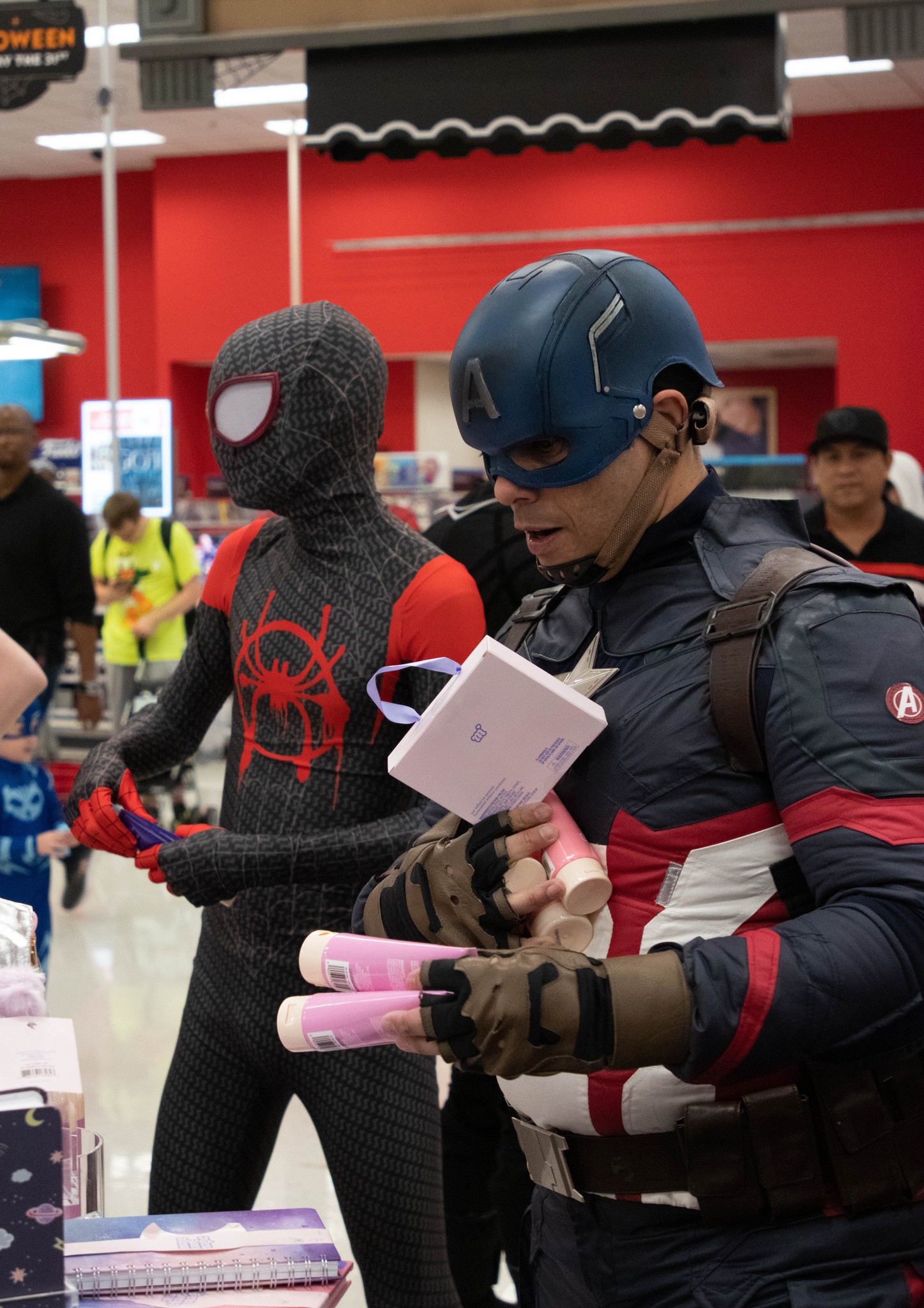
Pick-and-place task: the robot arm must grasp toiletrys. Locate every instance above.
[277,928,478,1056]
[500,787,613,953]
[113,804,236,908]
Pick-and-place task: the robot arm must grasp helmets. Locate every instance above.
[448,249,723,490]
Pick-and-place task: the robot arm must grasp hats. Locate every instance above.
[806,405,889,461]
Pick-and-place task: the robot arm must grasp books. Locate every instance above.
[0,1088,65,1308]
[63,1208,354,1308]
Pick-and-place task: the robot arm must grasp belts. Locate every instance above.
[514,1114,808,1204]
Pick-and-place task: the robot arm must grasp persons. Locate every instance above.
[350,249,924,1308]
[90,497,207,831]
[0,629,83,997]
[417,473,565,1308]
[781,404,924,631]
[0,400,105,752]
[65,300,489,1308]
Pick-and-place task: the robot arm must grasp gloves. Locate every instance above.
[130,825,244,909]
[62,747,156,857]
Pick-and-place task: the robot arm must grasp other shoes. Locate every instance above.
[58,845,92,908]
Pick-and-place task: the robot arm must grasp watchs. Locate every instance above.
[68,678,100,703]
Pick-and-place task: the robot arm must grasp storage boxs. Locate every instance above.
[389,636,608,825]
